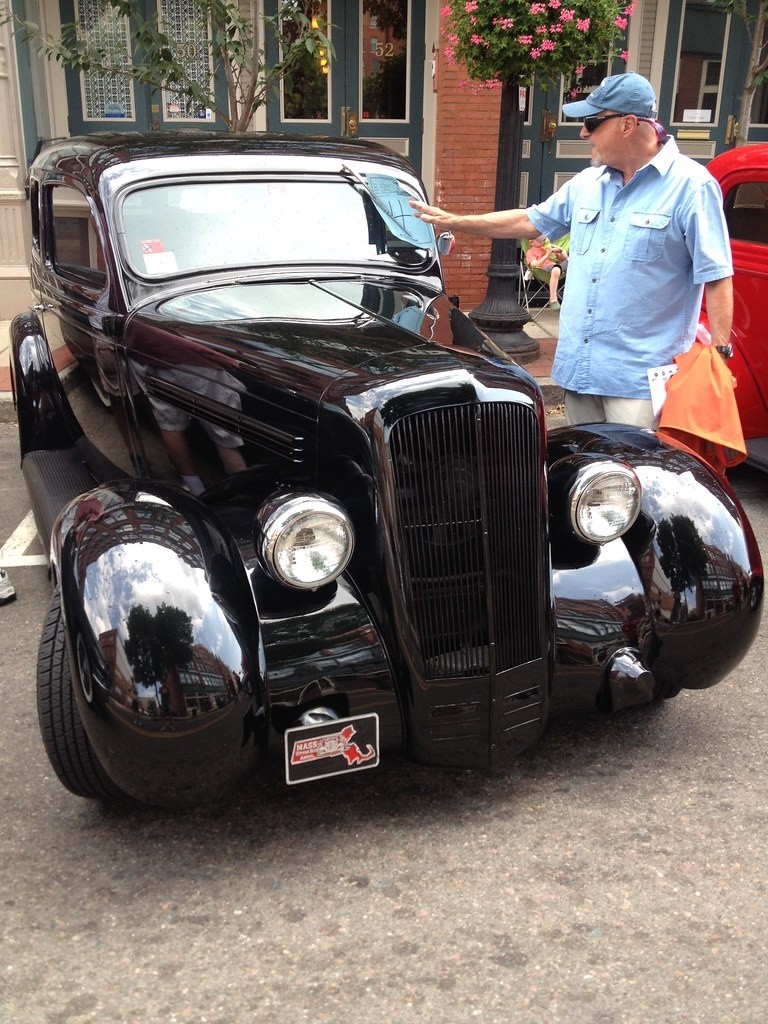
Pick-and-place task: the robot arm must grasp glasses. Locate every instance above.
[583,113,635,131]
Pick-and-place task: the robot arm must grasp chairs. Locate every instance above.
[515,235,572,327]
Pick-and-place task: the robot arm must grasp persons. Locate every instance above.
[407,69,735,439]
[526,233,568,312]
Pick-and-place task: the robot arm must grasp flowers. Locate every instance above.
[439,0,637,100]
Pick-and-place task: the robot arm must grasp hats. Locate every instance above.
[561,71,658,119]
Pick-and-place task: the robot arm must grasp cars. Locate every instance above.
[699,143,768,473]
[9,130,764,811]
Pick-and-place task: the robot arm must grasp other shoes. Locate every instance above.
[549,300,560,310]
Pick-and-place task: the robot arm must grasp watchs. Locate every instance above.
[713,342,734,359]
